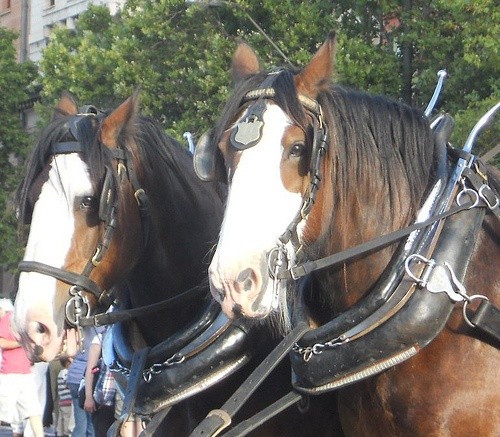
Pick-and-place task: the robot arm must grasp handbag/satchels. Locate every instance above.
[78,330,106,410]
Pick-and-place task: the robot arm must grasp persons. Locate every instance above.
[0,299,45,437]
[11,299,146,437]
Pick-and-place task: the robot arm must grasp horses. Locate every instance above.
[9,85,343,437]
[207,31,500,437]
